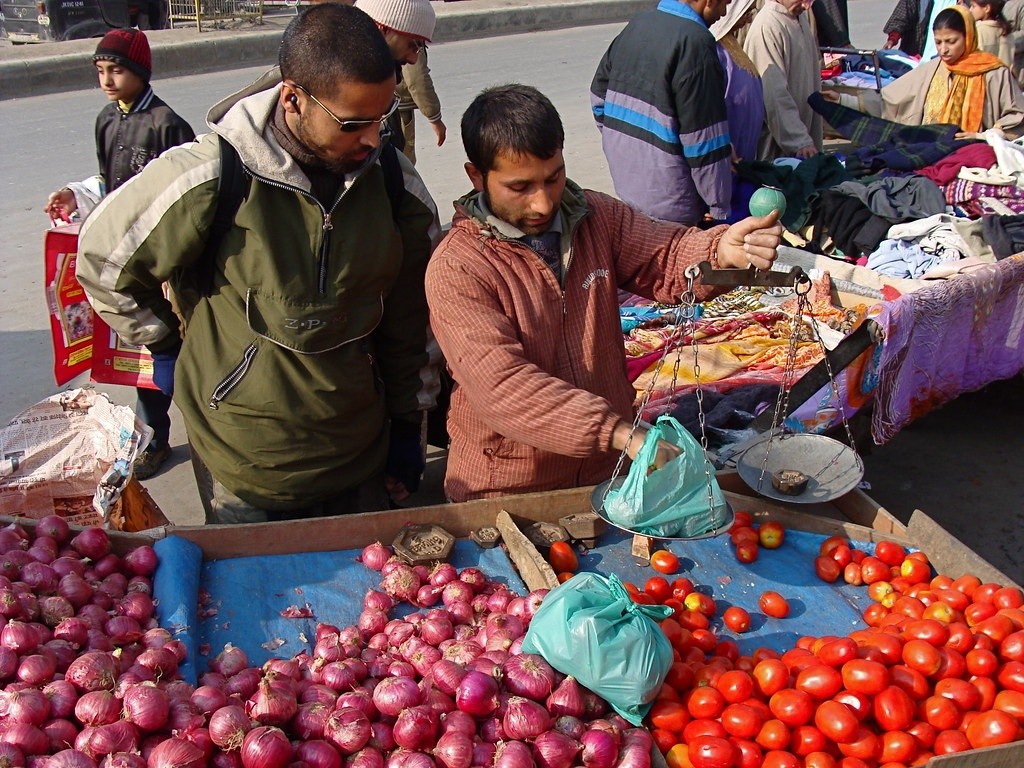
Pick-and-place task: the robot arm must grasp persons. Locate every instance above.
[425,83,782,504]
[742,0,823,159]
[354,0,436,85]
[75,3,445,524]
[811,0,855,49]
[820,7,1024,141]
[882,0,1024,70]
[709,0,764,163]
[590,0,732,226]
[394,45,447,167]
[45,28,199,479]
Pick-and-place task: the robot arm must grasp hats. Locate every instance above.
[355,1,434,40]
[92,26,156,82]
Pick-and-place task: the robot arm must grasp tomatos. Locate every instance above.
[547,511,1024,768]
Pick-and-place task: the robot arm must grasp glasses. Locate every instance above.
[294,82,403,133]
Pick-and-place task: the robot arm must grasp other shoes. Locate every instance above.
[130,441,174,483]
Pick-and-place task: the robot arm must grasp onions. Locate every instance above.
[0,514,652,768]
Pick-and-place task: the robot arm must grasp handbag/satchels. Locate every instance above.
[519,571,674,726]
[43,207,94,386]
[603,414,726,538]
